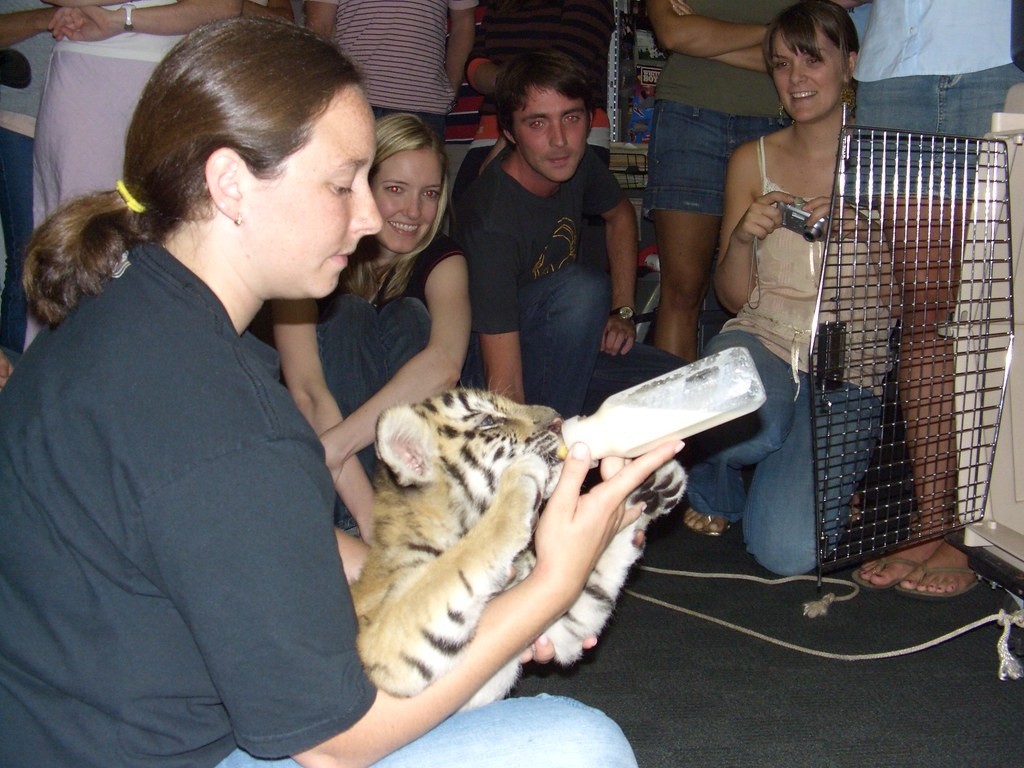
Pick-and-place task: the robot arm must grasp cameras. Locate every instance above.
[777,197,826,243]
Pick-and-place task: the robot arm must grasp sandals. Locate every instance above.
[895,561,979,598]
[848,457,886,528]
[682,507,730,536]
[852,554,920,590]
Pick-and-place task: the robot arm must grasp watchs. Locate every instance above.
[609,306,637,324]
[121,4,136,32]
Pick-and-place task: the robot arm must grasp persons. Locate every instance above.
[0,0,1024,598]
[0,16,686,768]
